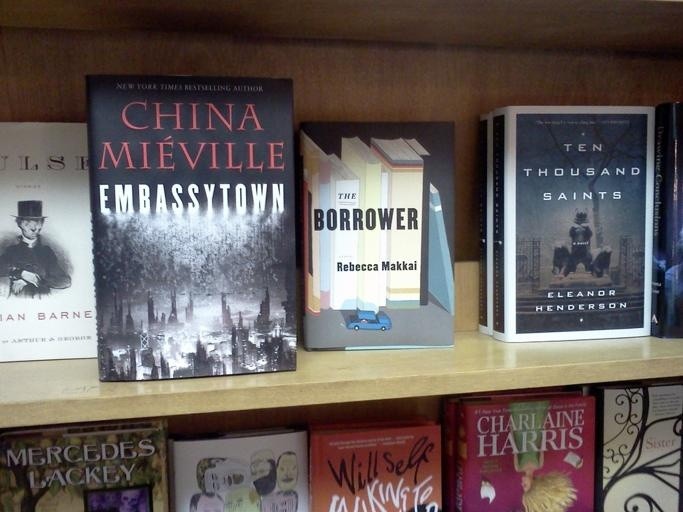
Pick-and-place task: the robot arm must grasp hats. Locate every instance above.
[11,201,48,219]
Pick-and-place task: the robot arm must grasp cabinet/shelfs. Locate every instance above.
[0,0,683,512]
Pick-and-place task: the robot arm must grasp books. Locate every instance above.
[299,120,454,351]
[477,104,652,343]
[2,386,682,512]
[649,101,683,341]
[1,73,295,382]
